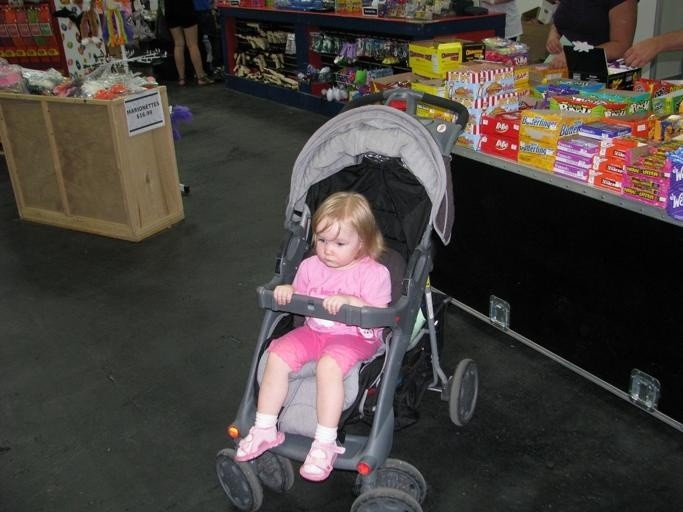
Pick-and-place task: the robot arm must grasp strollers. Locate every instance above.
[214,88,481,512]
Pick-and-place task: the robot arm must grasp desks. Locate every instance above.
[430,143,683,432]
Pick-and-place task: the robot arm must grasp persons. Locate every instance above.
[546,2,636,68]
[623,30,682,68]
[164,1,216,86]
[234,192,385,482]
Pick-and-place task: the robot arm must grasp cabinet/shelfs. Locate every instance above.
[215,6,509,117]
[0,85,187,246]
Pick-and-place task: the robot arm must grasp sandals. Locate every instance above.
[300,440,346,482]
[235,425,285,462]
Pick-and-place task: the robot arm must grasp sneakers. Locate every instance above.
[198,74,214,85]
[178,79,185,86]
